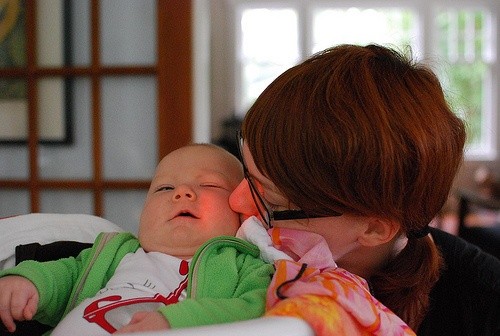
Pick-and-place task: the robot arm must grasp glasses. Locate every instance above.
[235,127,348,229]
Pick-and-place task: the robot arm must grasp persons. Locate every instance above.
[1,143,277,336]
[228,44,500,336]
